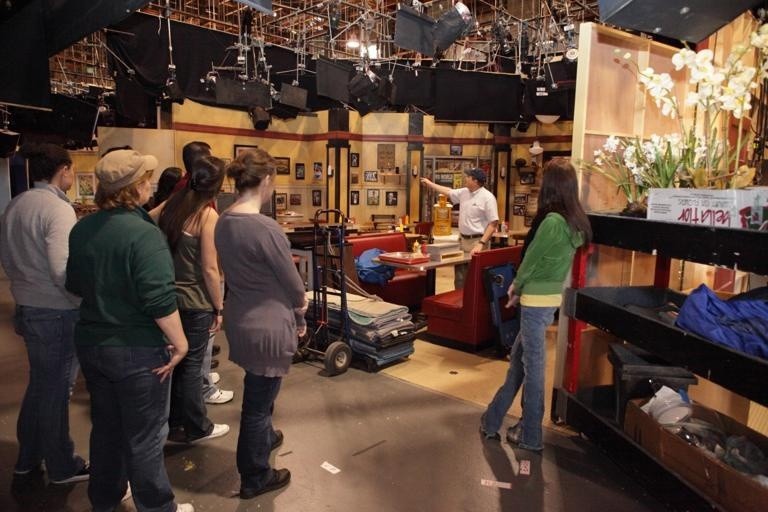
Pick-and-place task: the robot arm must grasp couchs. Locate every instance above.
[344,234,426,306]
[422,245,523,345]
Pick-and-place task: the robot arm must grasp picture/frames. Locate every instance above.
[350,153,397,205]
[520,171,535,185]
[450,144,462,156]
[513,193,528,216]
[234,144,322,210]
[75,172,96,199]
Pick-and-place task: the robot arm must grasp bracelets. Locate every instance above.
[478,240,486,245]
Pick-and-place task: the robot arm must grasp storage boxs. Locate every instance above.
[607,344,698,424]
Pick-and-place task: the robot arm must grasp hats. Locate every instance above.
[463,167,485,182]
[95,150,157,192]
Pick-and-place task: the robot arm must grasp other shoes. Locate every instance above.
[210,360,218,369]
[212,346,220,355]
[52,461,90,483]
[120,481,132,502]
[17,458,43,475]
[176,503,194,512]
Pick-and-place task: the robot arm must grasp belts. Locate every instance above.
[460,232,483,239]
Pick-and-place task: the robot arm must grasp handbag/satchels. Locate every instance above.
[354,248,394,287]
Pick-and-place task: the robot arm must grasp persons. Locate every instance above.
[478,157,592,452]
[418,167,499,290]
[387,193,397,204]
[140,141,235,446]
[0,141,91,486]
[212,147,309,500]
[64,149,193,511]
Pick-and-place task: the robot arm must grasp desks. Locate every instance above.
[371,250,472,296]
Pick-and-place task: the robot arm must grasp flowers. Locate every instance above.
[572,33,768,217]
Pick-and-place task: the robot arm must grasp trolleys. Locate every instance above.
[291,209,352,376]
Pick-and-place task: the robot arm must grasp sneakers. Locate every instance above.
[208,372,220,384]
[239,469,290,499]
[270,431,283,451]
[205,388,234,403]
[190,424,230,443]
[506,426,523,445]
